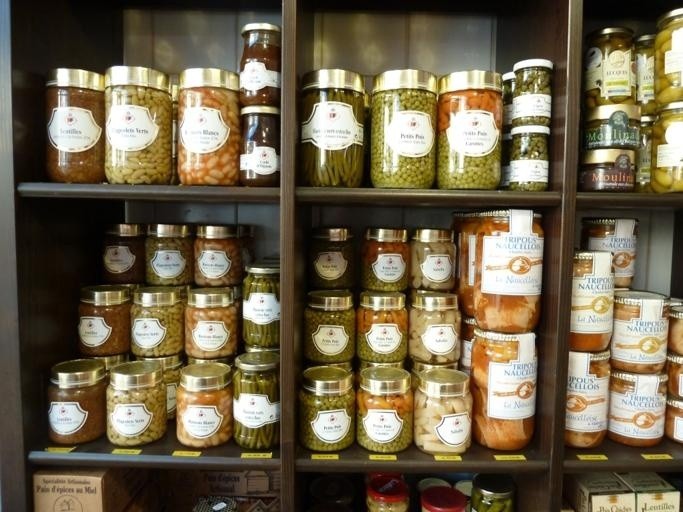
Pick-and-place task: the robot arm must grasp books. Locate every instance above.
[31,467,150,512]
[564,472,635,512]
[614,471,680,511]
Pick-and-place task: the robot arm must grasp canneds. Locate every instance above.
[565,217,683,448]
[364,476,514,512]
[300,209,545,450]
[581,6,682,192]
[300,58,553,190]
[45,22,280,185]
[44,225,282,448]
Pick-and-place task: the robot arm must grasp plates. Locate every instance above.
[2,1,681,512]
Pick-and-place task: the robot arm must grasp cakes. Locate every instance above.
[45,22,280,185]
[300,58,553,190]
[364,476,514,512]
[565,217,683,448]
[581,6,682,192]
